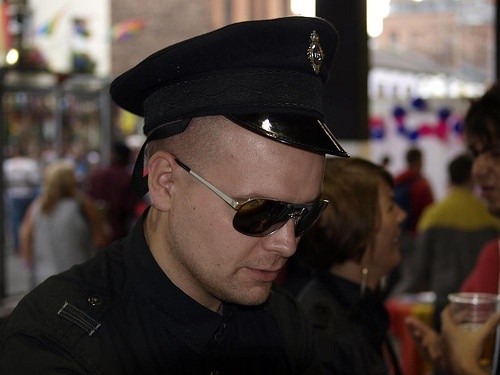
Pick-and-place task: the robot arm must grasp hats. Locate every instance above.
[108,16,350,159]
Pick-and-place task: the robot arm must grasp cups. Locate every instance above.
[447,291,499,375]
[405,291,438,329]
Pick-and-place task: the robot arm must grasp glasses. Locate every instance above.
[174,157,330,239]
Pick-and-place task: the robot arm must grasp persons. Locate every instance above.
[417,149,498,326]
[2,127,139,286]
[0,16,388,375]
[390,148,436,288]
[319,154,421,375]
[403,78,500,375]
